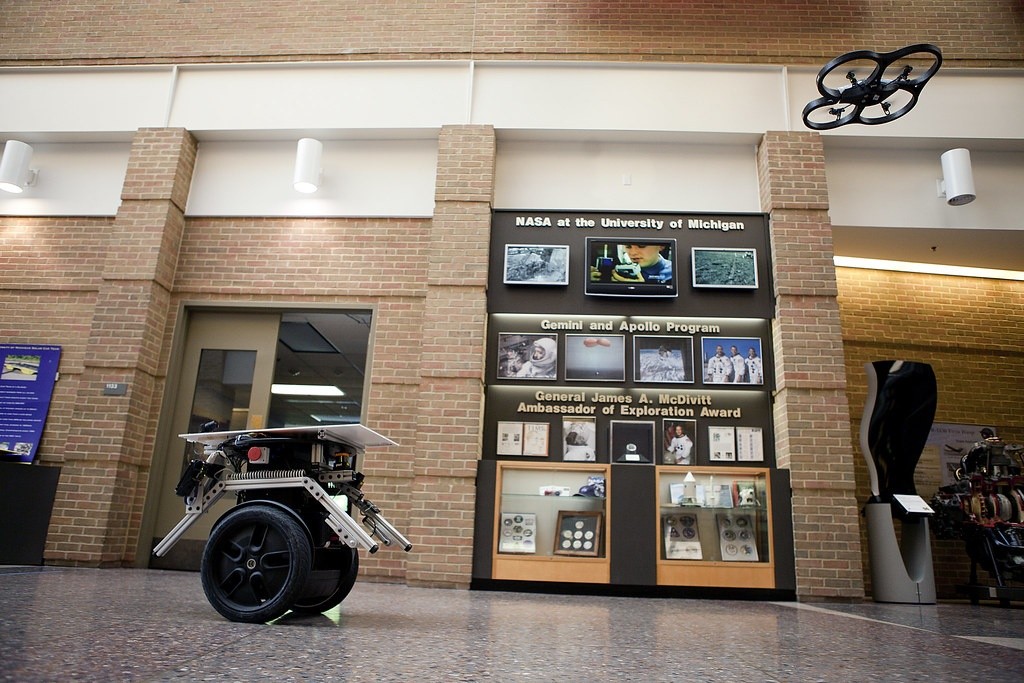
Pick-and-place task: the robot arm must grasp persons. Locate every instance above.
[744,348,762,383]
[708,346,732,382]
[728,346,746,383]
[507,338,557,377]
[667,425,692,464]
[591,244,672,284]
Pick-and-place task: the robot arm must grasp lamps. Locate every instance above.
[294,138,324,193]
[0,140,39,193]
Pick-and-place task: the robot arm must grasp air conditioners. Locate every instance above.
[936,147,977,206]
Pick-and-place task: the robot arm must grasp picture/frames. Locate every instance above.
[553,510,603,557]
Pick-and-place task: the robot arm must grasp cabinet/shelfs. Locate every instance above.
[492,460,611,586]
[655,464,775,590]
